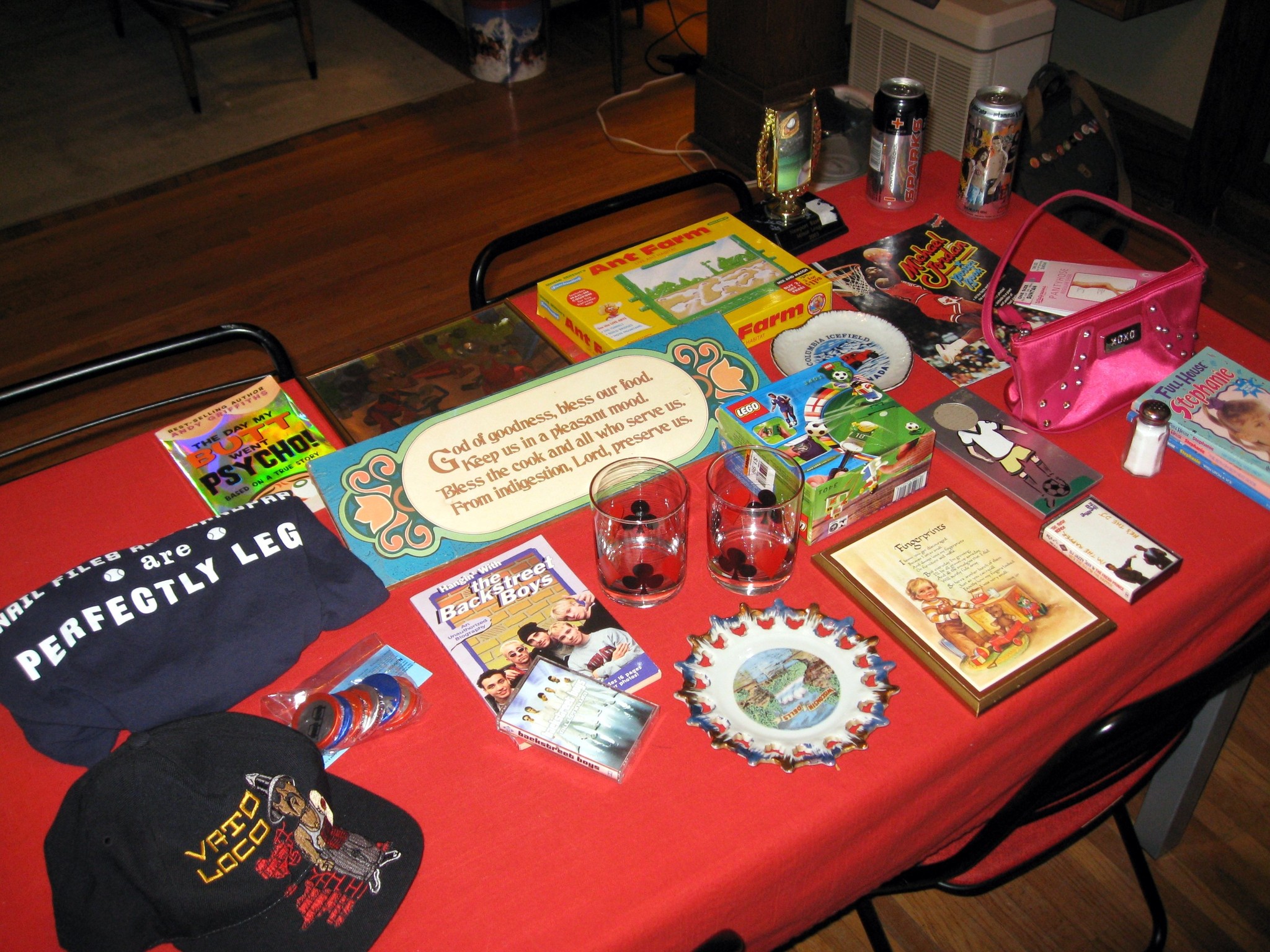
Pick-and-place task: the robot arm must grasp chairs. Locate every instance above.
[164,0,318,113]
[857,618,1270,952]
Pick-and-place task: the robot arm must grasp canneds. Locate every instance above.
[955,85,1023,219]
[865,77,929,210]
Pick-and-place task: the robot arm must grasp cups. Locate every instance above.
[704,444,806,596]
[588,456,690,611]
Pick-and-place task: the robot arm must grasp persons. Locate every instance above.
[757,393,845,488]
[960,125,1019,218]
[865,255,1062,386]
[1202,396,1270,460]
[1103,543,1173,586]
[474,585,653,761]
[1071,281,1128,296]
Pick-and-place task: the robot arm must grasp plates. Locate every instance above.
[769,310,915,391]
[672,597,900,774]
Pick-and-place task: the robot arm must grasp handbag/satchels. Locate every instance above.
[1013,63,1133,250]
[813,87,873,184]
[981,187,1209,432]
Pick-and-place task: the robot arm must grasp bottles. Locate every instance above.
[1120,399,1172,479]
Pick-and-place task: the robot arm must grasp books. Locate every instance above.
[408,532,662,752]
[1130,346,1270,511]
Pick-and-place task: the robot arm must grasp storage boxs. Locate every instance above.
[537,210,834,355]
[713,356,938,546]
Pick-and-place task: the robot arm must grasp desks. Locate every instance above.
[0,147,1270,952]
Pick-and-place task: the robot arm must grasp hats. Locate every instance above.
[44,712,424,952]
